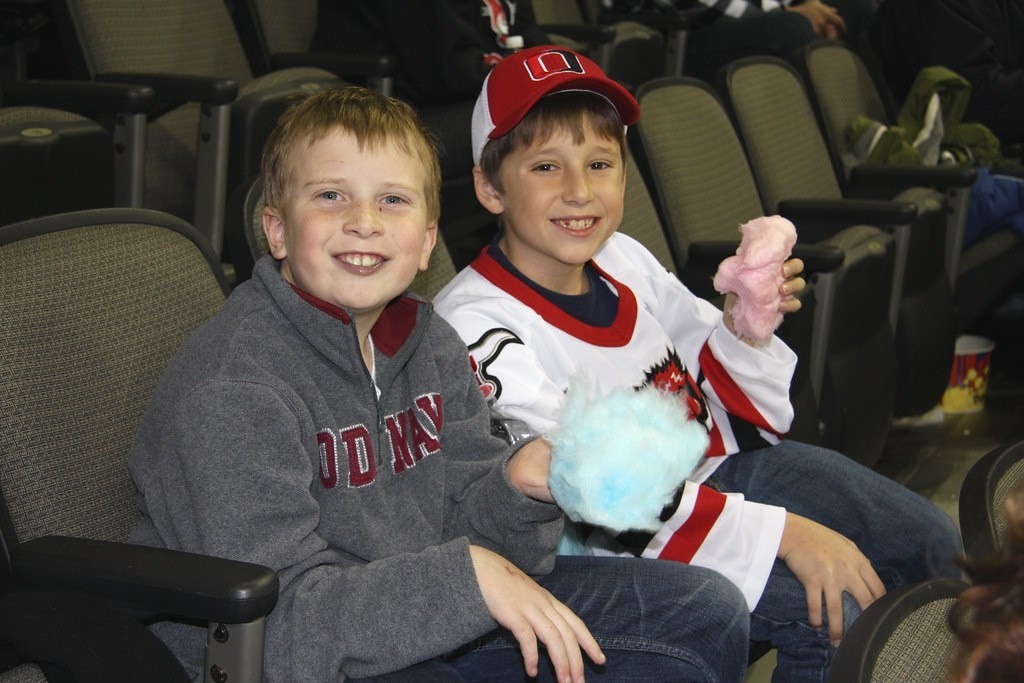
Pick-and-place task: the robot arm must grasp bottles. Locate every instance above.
[506,35,524,57]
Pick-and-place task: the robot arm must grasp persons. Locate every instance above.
[616,1,845,86]
[432,46,967,683]
[304,1,558,268]
[127,89,750,683]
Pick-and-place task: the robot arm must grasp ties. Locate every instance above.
[499,0,511,29]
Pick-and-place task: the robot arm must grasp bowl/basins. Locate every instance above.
[940,335,995,414]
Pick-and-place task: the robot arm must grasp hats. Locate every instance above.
[471,45,641,174]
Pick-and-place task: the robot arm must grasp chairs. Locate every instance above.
[0,0,1024,683]
